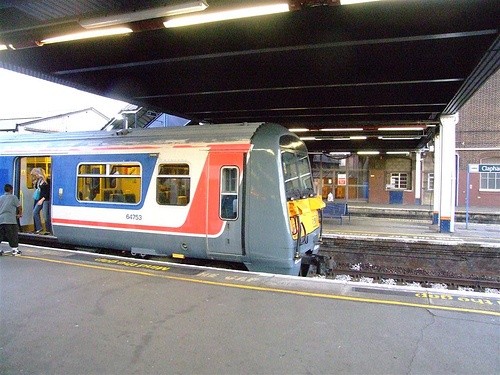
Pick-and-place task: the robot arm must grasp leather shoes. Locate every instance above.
[34,228,43,234]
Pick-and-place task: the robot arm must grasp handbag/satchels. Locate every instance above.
[33,178,43,200]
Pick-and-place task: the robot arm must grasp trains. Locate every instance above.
[0,122,327,276]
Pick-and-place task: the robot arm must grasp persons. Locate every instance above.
[0,184,22,257]
[30,167,44,231]
[32,166,52,236]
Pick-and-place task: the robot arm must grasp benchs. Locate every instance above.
[323,199,351,224]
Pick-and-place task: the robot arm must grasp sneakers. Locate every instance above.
[11,249,22,256]
